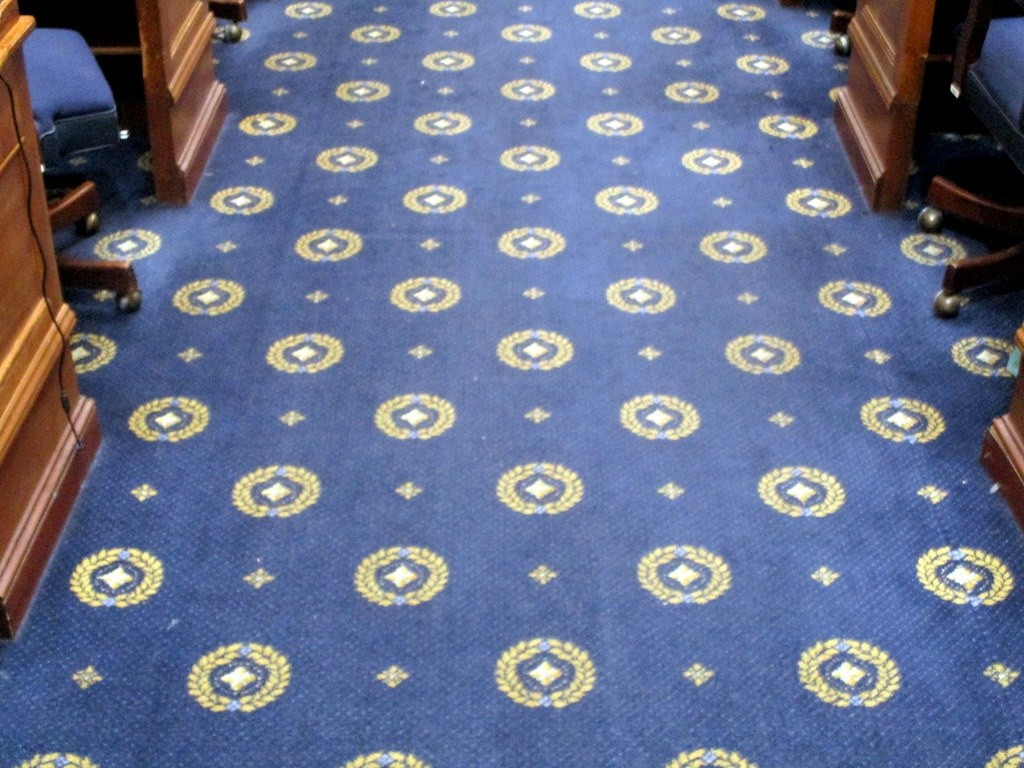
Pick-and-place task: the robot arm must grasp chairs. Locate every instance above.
[916,18,1024,318]
[23,27,143,312]
